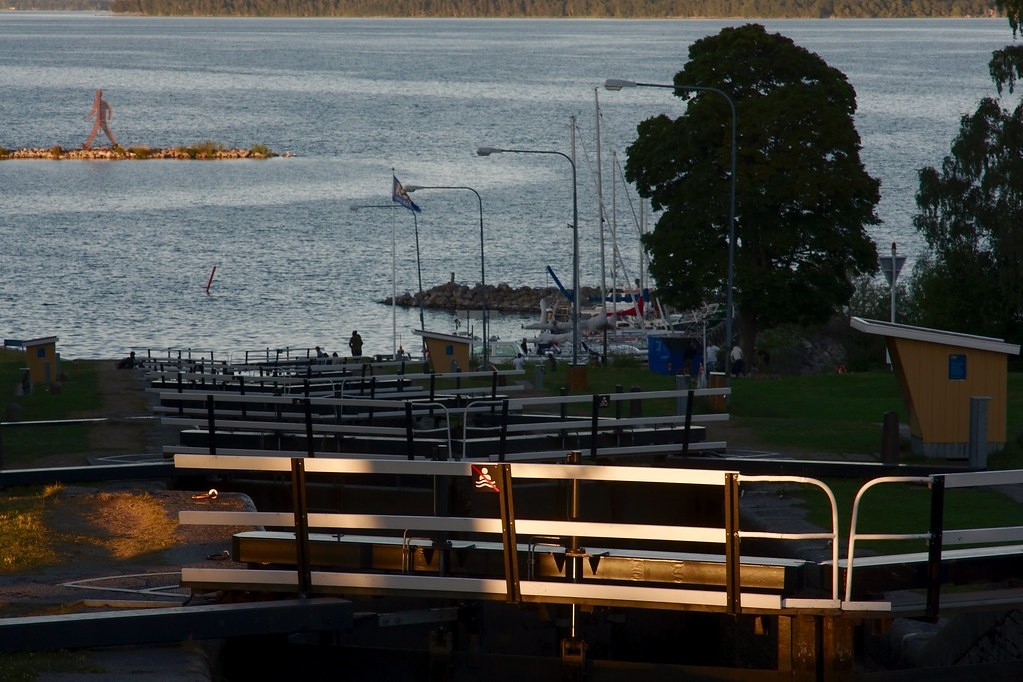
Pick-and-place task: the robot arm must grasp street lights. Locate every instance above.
[605,78,735,376]
[350,204,426,359]
[478,147,577,365]
[403,185,486,361]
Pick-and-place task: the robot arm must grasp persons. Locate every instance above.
[82,90,119,148]
[397,345,404,361]
[550,352,557,371]
[315,346,339,358]
[698,339,721,386]
[513,353,526,371]
[731,342,748,379]
[520,338,528,356]
[129,351,138,366]
[349,331,364,356]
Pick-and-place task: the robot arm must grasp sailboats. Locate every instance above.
[522,85,722,332]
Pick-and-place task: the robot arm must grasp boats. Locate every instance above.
[413,328,552,376]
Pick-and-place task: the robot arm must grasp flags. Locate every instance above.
[393,173,422,213]
